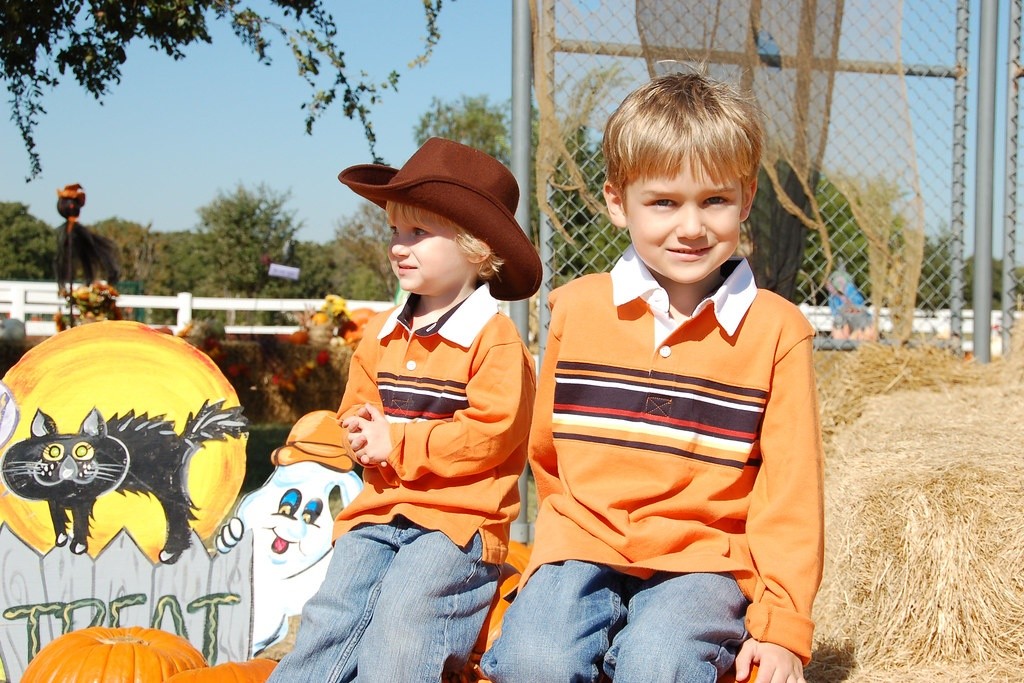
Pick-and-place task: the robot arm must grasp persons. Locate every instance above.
[264,138,543,683]
[481,74,826,683]
[826,271,877,339]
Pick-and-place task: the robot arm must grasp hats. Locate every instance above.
[339,138,543,302]
